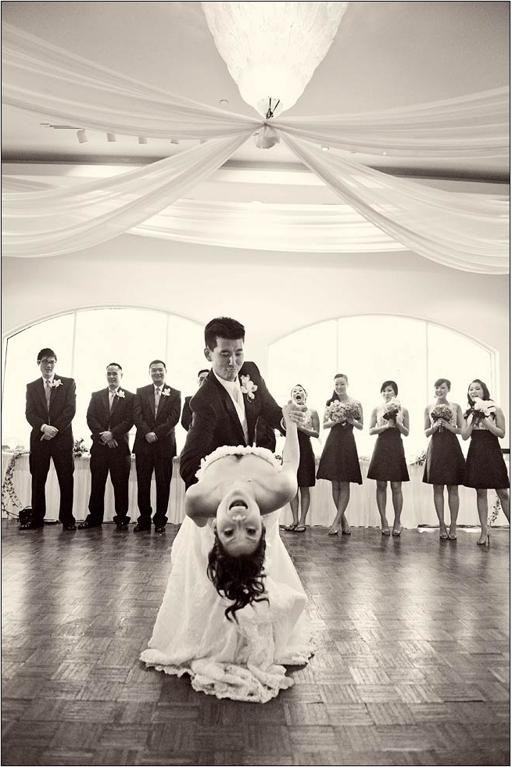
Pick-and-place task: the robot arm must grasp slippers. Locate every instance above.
[284,522,308,534]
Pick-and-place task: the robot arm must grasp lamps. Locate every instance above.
[198,2,350,123]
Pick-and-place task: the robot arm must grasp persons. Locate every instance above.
[77,363,134,531]
[461,379,510,544]
[316,374,363,535]
[19,348,77,530]
[133,360,180,532]
[179,317,312,492]
[283,384,320,533]
[424,378,464,540]
[369,381,410,537]
[180,369,210,431]
[139,392,319,705]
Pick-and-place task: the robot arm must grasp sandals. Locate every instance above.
[440,525,449,540]
[450,524,457,540]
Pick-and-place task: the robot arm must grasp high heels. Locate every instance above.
[476,528,492,546]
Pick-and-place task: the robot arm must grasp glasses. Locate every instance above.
[40,358,58,367]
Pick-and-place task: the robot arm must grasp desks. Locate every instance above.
[411,449,510,531]
[2,445,419,534]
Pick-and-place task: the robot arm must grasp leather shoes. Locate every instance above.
[19,518,45,530]
[77,519,100,530]
[344,523,351,535]
[393,522,404,537]
[60,518,75,532]
[154,525,164,532]
[133,524,151,533]
[380,521,390,536]
[328,522,340,535]
[115,523,128,530]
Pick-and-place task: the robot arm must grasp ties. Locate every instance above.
[44,378,52,426]
[153,387,159,421]
[108,390,115,430]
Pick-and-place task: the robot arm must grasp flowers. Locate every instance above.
[466,396,499,428]
[115,389,125,399]
[239,373,259,400]
[51,378,62,387]
[324,399,361,427]
[430,405,454,433]
[383,401,399,424]
[161,388,171,397]
